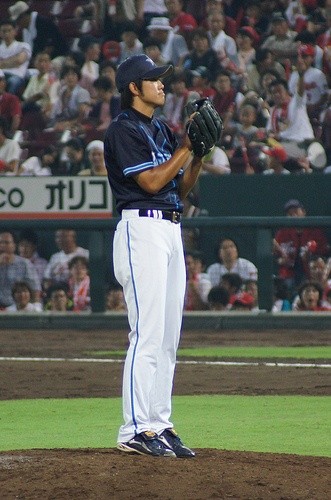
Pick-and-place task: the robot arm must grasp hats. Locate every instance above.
[10,2,29,20]
[283,200,302,210]
[114,54,173,93]
[146,16,173,31]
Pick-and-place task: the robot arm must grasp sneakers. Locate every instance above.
[159,428,195,457]
[117,431,176,458]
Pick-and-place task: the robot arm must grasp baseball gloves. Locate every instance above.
[185,96,223,159]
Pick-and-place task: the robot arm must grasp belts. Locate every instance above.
[139,210,183,224]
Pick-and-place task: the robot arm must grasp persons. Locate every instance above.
[103,55,225,459]
[0,0,331,315]
